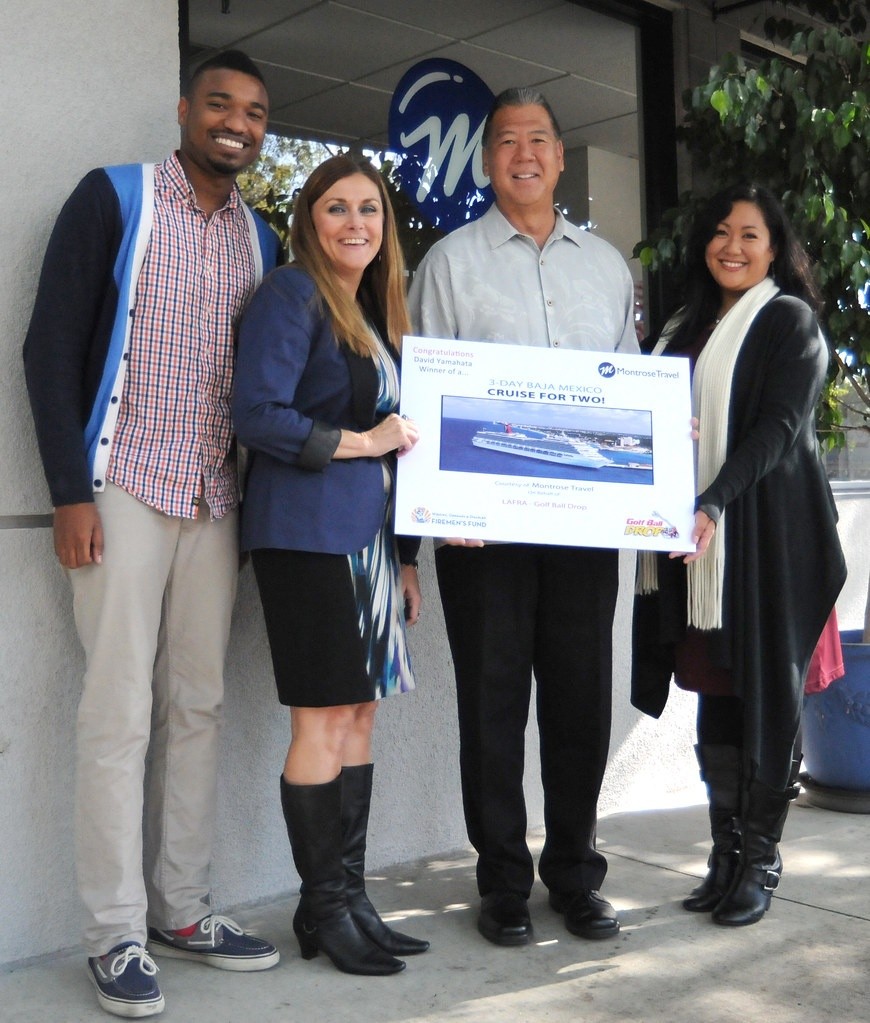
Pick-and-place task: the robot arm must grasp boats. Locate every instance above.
[471,422,611,470]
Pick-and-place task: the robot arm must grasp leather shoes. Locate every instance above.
[476,886,534,946]
[549,886,621,940]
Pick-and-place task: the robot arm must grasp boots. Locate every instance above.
[682,743,746,912]
[712,756,801,925]
[339,762,430,957]
[279,770,407,975]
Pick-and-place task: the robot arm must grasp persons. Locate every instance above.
[244,152,433,975]
[392,82,658,950]
[27,41,300,1015]
[647,171,852,931]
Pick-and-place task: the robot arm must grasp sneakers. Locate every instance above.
[146,913,280,971]
[85,938,165,1017]
